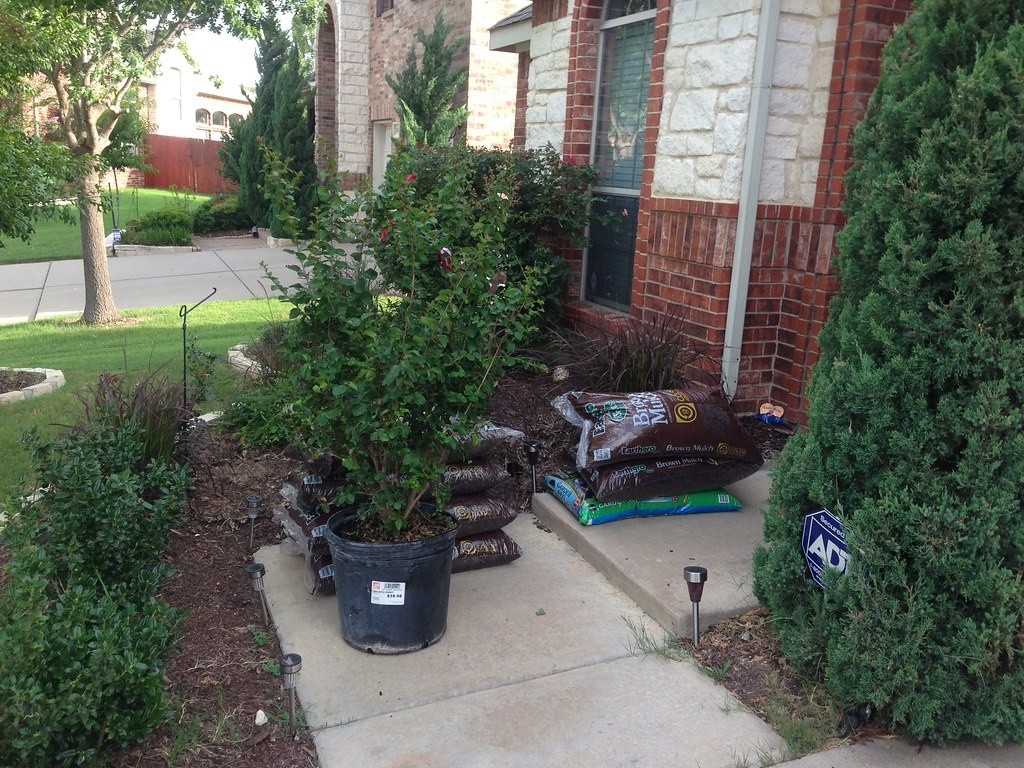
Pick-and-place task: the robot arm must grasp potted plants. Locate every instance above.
[214,147,547,655]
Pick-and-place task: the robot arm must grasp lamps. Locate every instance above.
[684,566,707,646]
[245,492,301,736]
[524,440,541,493]
